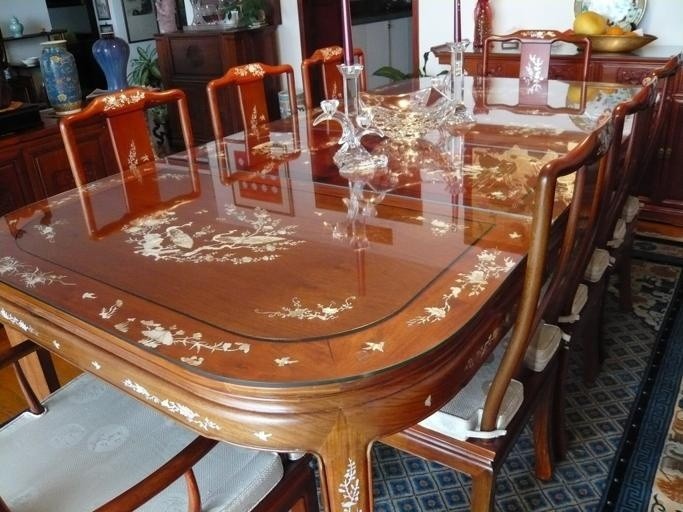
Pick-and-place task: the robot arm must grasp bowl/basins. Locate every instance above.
[20,55,39,67]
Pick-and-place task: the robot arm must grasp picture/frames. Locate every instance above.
[121,0,160,44]
[100,25,113,33]
[95,0,111,20]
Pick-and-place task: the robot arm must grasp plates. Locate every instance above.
[564,32,659,54]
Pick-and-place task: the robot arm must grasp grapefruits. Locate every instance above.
[574,11,608,36]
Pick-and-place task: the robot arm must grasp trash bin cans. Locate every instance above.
[278,89,305,119]
